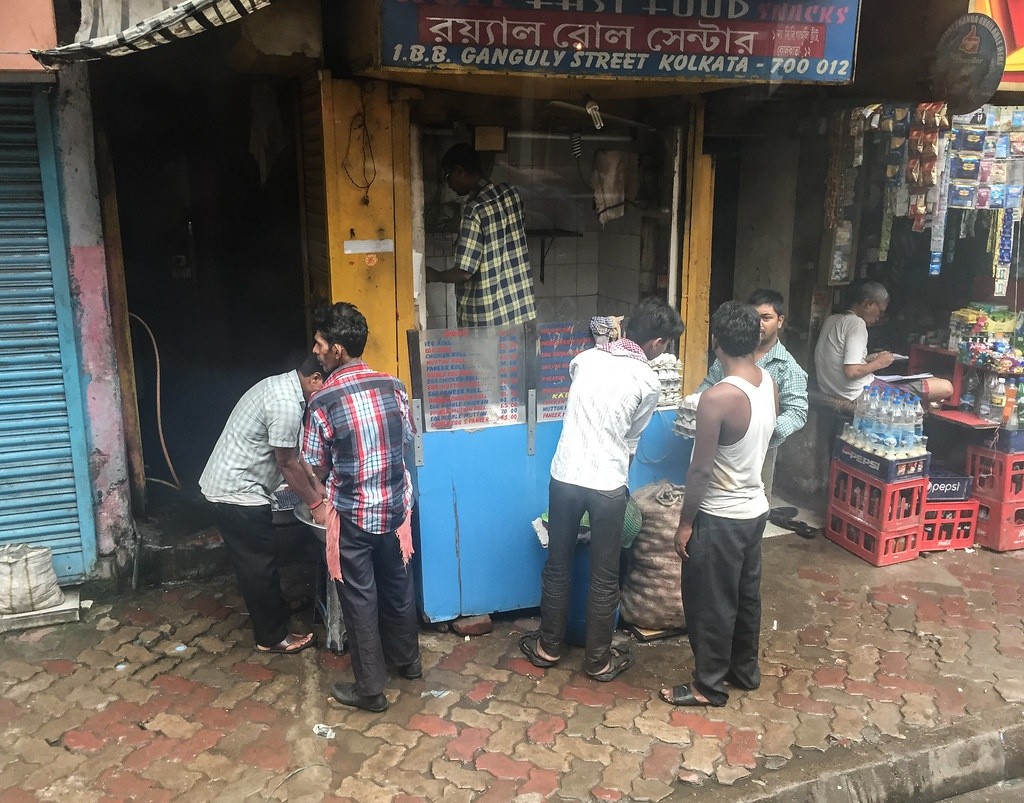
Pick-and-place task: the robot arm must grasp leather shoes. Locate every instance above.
[405,658,422,678]
[331,680,388,711]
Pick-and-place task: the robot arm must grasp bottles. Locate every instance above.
[958,366,1024,430]
[852,386,923,451]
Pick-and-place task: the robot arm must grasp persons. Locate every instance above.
[300,303,425,713]
[657,302,780,709]
[815,284,954,405]
[519,294,683,684]
[697,289,809,516]
[198,349,329,654]
[425,142,537,330]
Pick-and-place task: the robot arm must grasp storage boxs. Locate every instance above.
[919,498,980,551]
[828,458,929,532]
[824,504,926,567]
[925,465,974,501]
[966,445,1024,503]
[983,426,1024,453]
[832,426,932,484]
[970,493,1024,551]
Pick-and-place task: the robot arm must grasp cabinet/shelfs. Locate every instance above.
[904,344,1024,431]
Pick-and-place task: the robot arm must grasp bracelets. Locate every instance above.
[308,499,323,511]
[307,474,316,479]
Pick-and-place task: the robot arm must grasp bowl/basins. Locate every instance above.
[293,500,326,546]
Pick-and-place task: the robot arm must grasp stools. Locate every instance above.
[826,409,854,459]
[313,538,348,655]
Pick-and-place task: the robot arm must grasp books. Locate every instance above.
[876,372,934,383]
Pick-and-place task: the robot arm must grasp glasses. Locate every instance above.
[874,297,887,316]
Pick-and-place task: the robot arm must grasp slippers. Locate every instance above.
[591,644,635,681]
[254,633,318,654]
[659,682,727,707]
[724,668,760,690]
[518,631,554,667]
